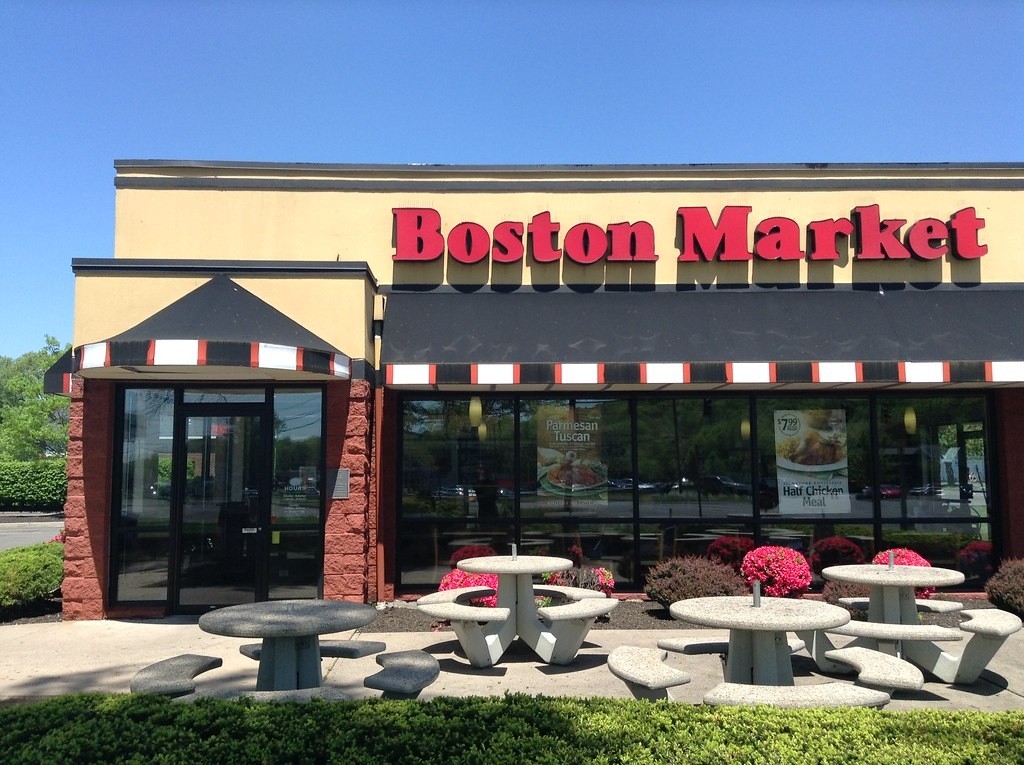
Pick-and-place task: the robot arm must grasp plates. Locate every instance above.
[540,477,608,497]
[547,474,608,491]
[776,453,848,471]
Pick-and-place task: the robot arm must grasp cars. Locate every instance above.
[864,482,941,500]
[432,485,515,502]
[602,474,752,497]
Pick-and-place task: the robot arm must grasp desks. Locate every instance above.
[454,554,575,674]
[816,563,966,687]
[198,598,382,688]
[666,595,852,697]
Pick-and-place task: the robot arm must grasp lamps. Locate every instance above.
[741,415,751,442]
[903,404,917,436]
[468,395,488,441]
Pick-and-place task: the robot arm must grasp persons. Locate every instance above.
[418,482,433,499]
[473,469,500,531]
[918,485,943,532]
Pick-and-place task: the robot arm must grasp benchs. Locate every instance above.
[534,582,619,597]
[238,631,387,668]
[218,681,356,704]
[411,584,496,606]
[705,680,892,709]
[827,620,961,658]
[833,593,965,659]
[824,643,933,691]
[606,644,692,702]
[119,646,217,702]
[956,604,1022,696]
[837,591,969,611]
[360,646,446,699]
[521,595,621,664]
[419,602,512,668]
[657,634,805,656]
